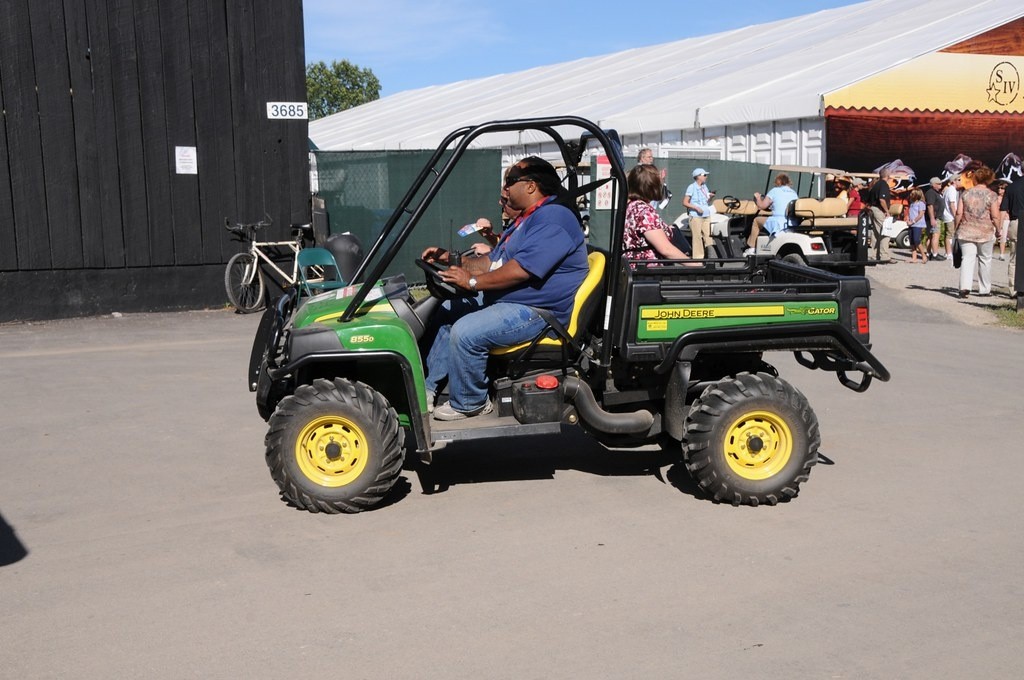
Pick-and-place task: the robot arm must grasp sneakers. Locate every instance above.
[425,388,436,412]
[433,394,493,421]
[742,248,756,258]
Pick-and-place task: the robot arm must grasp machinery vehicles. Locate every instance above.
[242,116,894,513]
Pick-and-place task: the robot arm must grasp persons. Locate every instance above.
[742,174,800,258]
[621,148,715,268]
[421,156,590,422]
[834,152,1024,299]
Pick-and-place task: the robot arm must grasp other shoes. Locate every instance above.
[929,253,947,261]
[959,289,970,299]
[886,258,898,264]
[922,257,928,263]
[944,253,953,259]
[1009,293,1018,300]
[905,259,918,263]
[999,255,1005,261]
[979,293,992,297]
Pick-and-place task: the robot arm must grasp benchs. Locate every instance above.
[788,195,859,230]
[712,199,758,221]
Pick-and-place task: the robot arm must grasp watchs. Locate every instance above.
[468,275,478,293]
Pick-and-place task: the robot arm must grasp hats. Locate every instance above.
[930,177,941,187]
[949,175,961,183]
[836,174,852,184]
[692,168,709,178]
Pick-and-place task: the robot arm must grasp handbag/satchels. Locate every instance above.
[658,183,672,210]
[952,238,962,269]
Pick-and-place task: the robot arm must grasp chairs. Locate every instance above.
[490,251,606,375]
[297,247,349,312]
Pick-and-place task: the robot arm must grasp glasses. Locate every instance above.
[956,179,961,181]
[701,174,706,177]
[998,186,1005,190]
[935,183,942,185]
[506,176,533,187]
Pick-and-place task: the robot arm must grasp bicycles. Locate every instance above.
[222,216,331,314]
